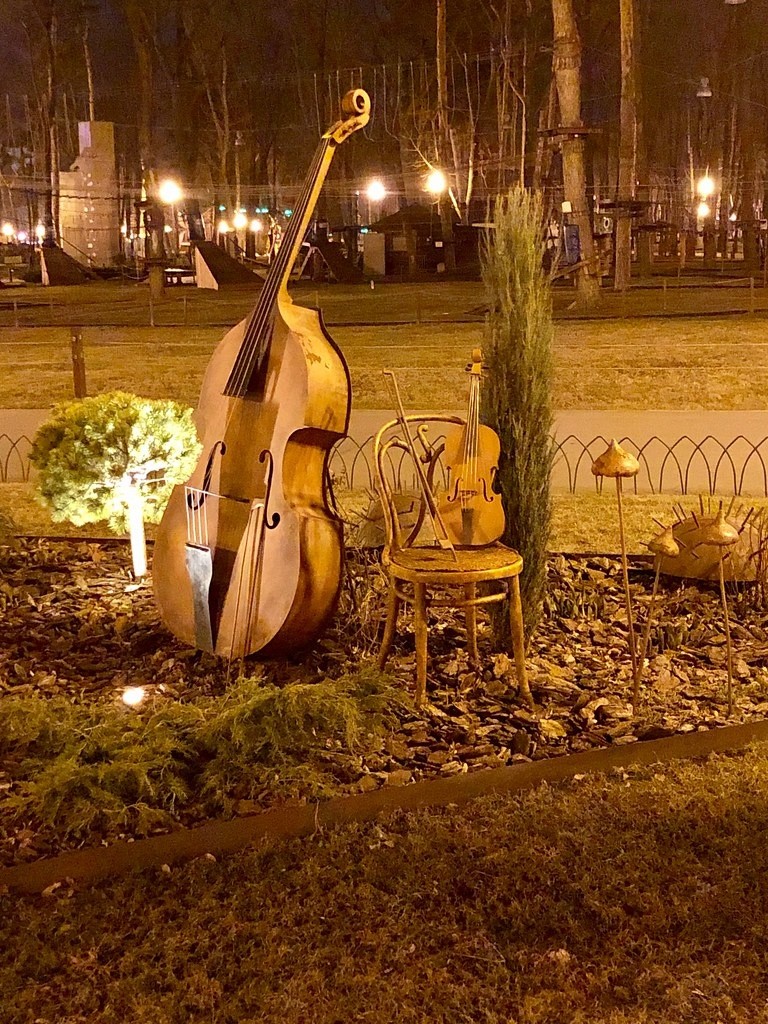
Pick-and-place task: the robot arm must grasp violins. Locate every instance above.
[432,347,508,551]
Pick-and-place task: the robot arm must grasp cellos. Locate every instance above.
[152,85,372,683]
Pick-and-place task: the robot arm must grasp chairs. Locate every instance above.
[374,414,533,713]
